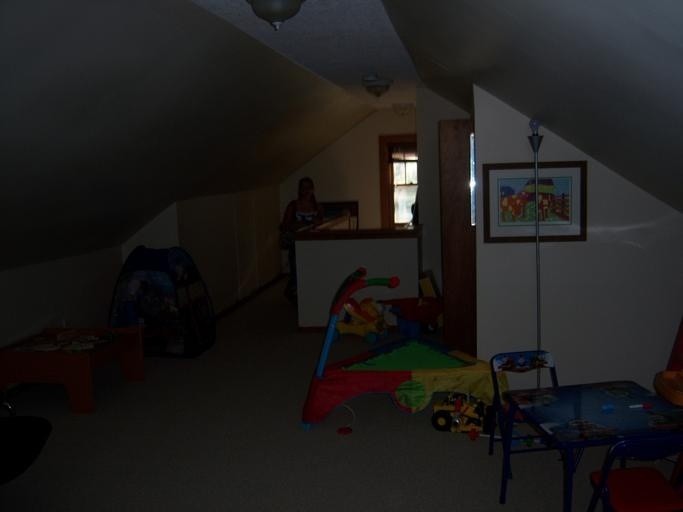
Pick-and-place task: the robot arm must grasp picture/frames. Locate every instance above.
[483,161,586,240]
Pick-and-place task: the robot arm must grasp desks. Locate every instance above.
[498,380,683,512]
[1,327,146,412]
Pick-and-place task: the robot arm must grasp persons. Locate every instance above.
[280,177,322,300]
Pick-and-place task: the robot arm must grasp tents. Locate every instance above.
[107,241,217,360]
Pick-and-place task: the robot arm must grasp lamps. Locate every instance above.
[246,0,305,31]
[362,74,393,98]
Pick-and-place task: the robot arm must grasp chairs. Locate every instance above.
[586,430,683,512]
[1,399,52,485]
[488,350,559,479]
